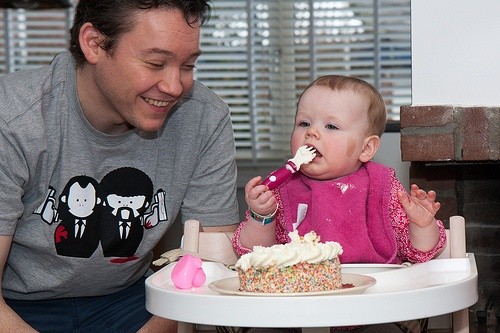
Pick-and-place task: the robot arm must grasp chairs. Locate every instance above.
[144,215,478,333]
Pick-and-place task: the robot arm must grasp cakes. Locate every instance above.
[235,230,343,294]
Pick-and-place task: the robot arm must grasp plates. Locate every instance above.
[207,273,377,296]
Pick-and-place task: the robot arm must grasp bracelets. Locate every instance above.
[250,207,280,224]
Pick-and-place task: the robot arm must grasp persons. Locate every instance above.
[232,74,447,263]
[0,0,239,333]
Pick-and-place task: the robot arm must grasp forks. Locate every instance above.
[261,145,317,191]
